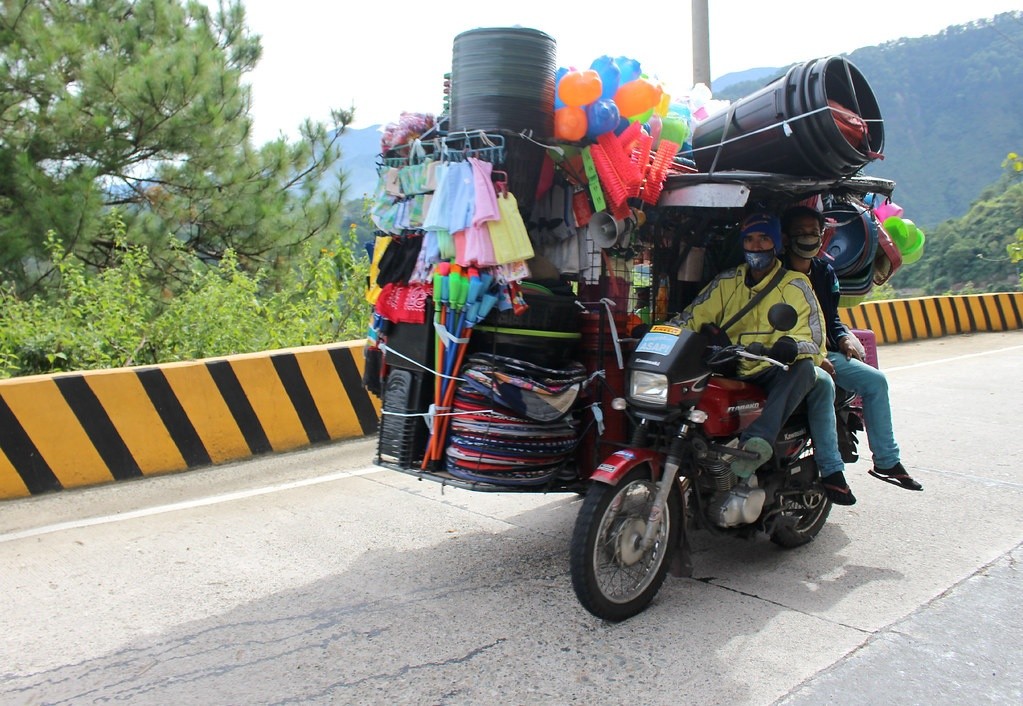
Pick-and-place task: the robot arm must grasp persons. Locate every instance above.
[782,205,924,505]
[632,208,828,478]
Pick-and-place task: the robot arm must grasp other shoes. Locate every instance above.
[730,437,773,479]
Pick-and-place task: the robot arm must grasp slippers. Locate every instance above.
[821,475,857,505]
[868,469,924,491]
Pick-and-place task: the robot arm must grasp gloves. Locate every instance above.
[631,323,653,339]
[769,336,798,366]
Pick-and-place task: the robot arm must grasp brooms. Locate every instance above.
[420,261,499,471]
[589,119,680,221]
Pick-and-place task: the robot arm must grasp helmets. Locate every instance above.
[740,213,782,257]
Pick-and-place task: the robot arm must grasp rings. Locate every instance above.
[832,370,835,375]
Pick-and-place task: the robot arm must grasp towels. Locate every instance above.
[453,230,472,268]
[425,231,443,264]
[447,158,476,236]
[475,221,498,266]
[374,158,443,207]
[373,281,434,325]
[525,178,591,277]
[363,230,428,306]
[471,157,501,230]
[422,160,450,232]
[463,227,479,263]
[367,192,433,236]
[436,231,456,260]
[486,191,536,265]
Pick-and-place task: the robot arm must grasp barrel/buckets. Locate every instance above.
[672,242,706,282]
[688,53,886,179]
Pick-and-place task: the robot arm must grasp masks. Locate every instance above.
[787,233,822,259]
[743,246,776,271]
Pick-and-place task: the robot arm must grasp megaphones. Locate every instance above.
[587,206,647,249]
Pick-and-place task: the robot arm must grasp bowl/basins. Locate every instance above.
[820,200,926,295]
